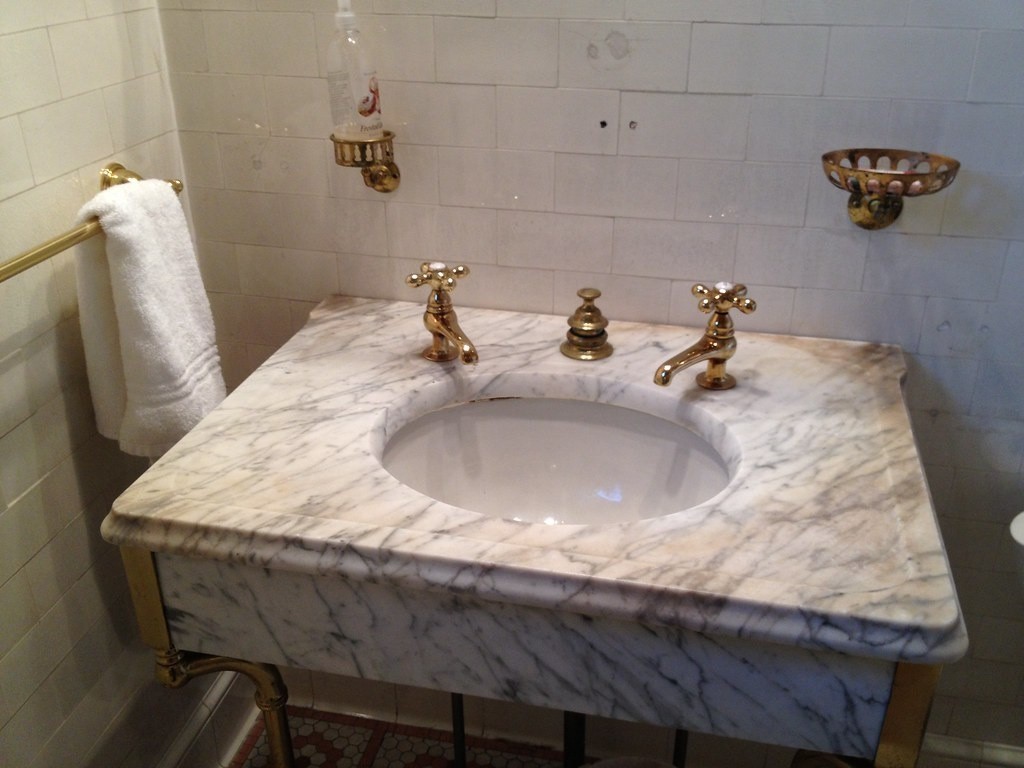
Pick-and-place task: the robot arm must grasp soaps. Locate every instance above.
[849,168,922,193]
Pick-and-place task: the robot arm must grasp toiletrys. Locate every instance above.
[324,1,383,165]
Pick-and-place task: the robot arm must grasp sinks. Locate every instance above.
[365,364,746,529]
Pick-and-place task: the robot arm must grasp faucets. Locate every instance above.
[405,262,478,363]
[653,281,758,391]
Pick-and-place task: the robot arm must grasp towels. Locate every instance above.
[71,175,228,458]
